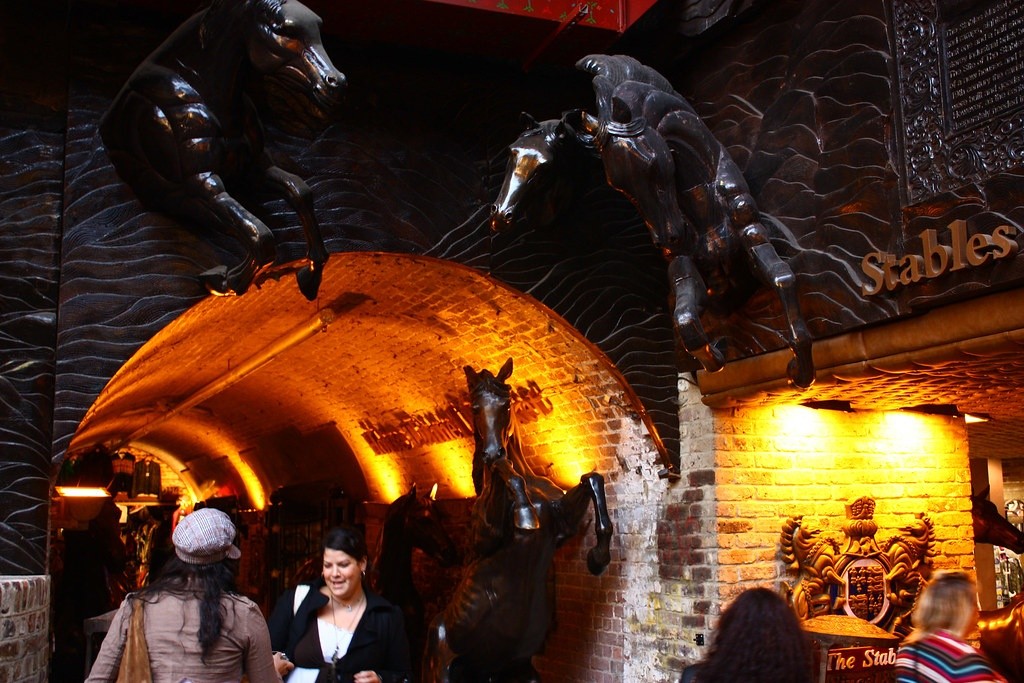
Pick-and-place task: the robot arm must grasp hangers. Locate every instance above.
[144,456,152,462]
[118,450,125,454]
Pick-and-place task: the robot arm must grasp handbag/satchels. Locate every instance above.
[115,591,153,683]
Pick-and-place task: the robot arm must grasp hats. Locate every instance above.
[171,508,241,565]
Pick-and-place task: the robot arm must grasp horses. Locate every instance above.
[488,52,818,393]
[779,511,937,630]
[94,1,352,301]
[972,483,1024,555]
[282,355,615,683]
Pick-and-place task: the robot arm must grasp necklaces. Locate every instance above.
[332,597,354,609]
[331,595,364,668]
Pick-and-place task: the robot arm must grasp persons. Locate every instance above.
[681,588,814,683]
[893,572,1010,683]
[84,507,410,683]
